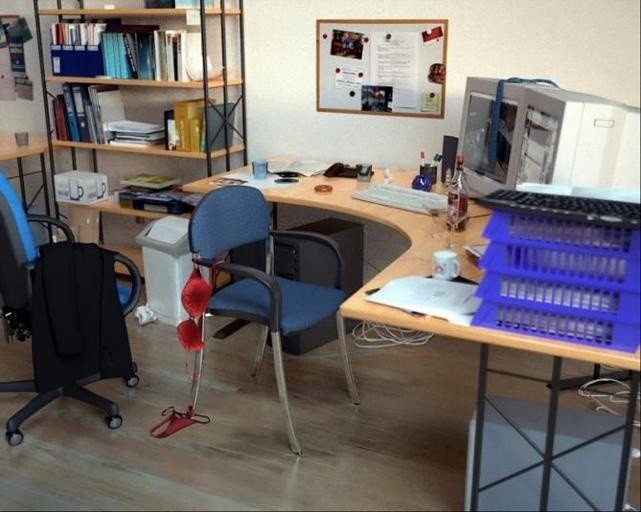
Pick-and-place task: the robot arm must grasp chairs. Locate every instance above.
[0,171,141,449]
[184,182,362,458]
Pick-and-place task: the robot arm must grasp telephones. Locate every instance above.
[325,162,357,179]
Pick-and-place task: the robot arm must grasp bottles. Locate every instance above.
[446,154,468,232]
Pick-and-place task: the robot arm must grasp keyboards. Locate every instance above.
[351,183,450,215]
[474,189,641,230]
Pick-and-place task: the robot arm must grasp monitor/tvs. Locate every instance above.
[457,77,565,199]
[511,87,641,191]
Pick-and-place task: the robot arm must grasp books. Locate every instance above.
[52,94,71,142]
[50,20,212,81]
[120,172,182,189]
[84,84,166,149]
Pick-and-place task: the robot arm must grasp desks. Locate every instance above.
[181,158,640,510]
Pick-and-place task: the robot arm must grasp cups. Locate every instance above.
[14,131,29,147]
[432,250,461,281]
[357,163,372,183]
[419,163,437,186]
[252,161,269,180]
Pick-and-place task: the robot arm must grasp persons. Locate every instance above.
[360,89,386,103]
[331,30,365,59]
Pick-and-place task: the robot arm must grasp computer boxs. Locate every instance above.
[464,395,632,512]
[266,216,363,355]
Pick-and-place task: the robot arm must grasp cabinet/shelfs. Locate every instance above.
[30,0,252,294]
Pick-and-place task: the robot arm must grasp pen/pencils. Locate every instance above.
[420,152,442,168]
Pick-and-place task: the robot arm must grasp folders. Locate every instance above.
[61,84,90,143]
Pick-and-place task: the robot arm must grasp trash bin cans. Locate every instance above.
[136,214,209,328]
